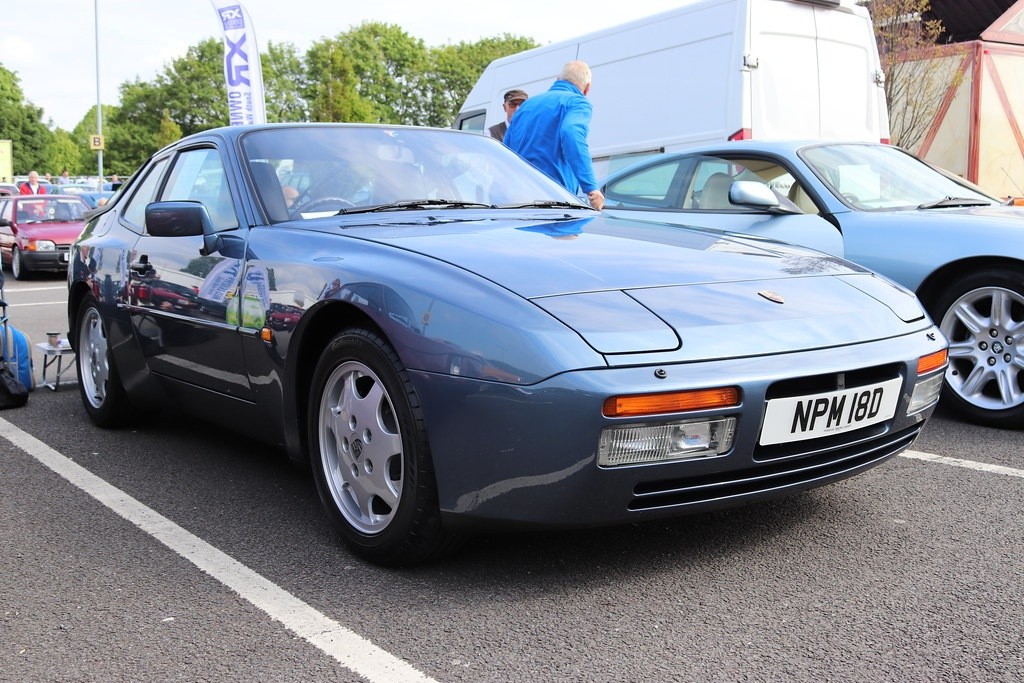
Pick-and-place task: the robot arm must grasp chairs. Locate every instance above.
[16,211,29,221]
[701,173,734,211]
[216,162,291,230]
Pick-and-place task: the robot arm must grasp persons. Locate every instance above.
[110,174,119,183]
[514,217,596,241]
[19,170,47,219]
[1,177,7,183]
[45,173,54,184]
[494,61,606,211]
[473,89,529,203]
[57,171,69,186]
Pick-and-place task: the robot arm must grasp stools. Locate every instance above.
[37,339,79,391]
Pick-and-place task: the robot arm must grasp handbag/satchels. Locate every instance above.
[0,367,29,411]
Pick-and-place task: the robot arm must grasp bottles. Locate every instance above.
[46,332,61,348]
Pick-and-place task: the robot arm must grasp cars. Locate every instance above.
[66,122,963,559]
[0,176,127,210]
[0,196,98,277]
[586,138,1023,420]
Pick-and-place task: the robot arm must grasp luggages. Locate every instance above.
[0,286,35,392]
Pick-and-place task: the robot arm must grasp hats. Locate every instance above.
[504,89,528,101]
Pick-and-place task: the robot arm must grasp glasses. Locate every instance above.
[508,102,523,108]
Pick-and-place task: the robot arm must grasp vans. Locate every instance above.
[451,0,903,198]
